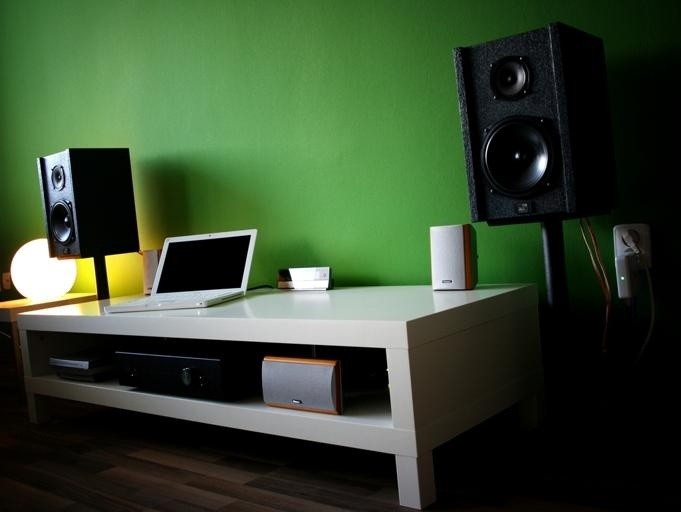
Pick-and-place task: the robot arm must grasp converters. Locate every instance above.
[614,256,633,299]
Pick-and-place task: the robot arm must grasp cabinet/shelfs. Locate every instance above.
[0,288,97,416]
[15,281,546,512]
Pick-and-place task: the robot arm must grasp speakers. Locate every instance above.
[429,223,479,291]
[262,355,343,415]
[451,21,622,227]
[143,248,163,295]
[36,147,140,260]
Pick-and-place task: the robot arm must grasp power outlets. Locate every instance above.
[611,223,652,273]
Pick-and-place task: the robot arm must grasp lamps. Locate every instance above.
[10,237,77,298]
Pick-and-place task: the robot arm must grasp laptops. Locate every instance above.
[104,228,258,313]
